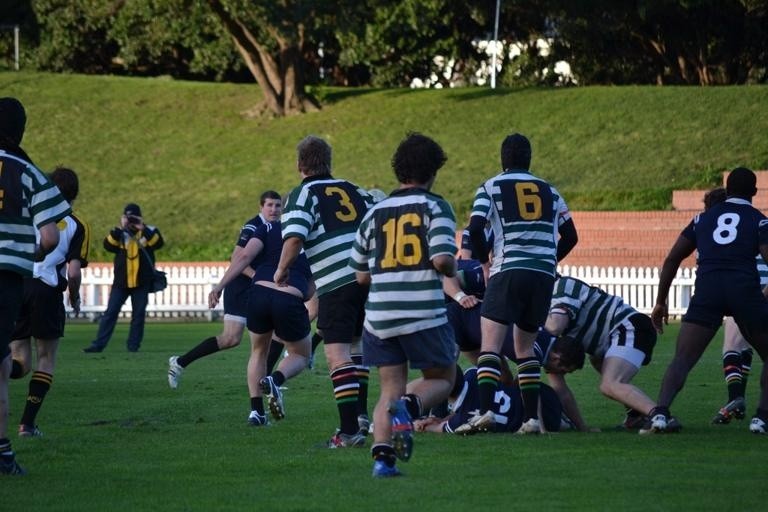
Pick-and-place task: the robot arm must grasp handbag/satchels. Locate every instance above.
[150,269,167,293]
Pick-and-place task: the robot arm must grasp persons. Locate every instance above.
[10,167,93,438]
[455,134,578,435]
[636,165,767,435]
[305,226,601,432]
[273,136,376,447]
[167,191,280,389]
[544,271,682,434]
[692,188,768,425]
[208,219,316,428]
[348,133,458,478]
[83,203,165,353]
[0,95,73,477]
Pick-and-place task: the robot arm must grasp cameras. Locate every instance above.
[125,217,142,236]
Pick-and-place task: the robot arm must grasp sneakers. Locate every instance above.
[168,355,185,391]
[710,396,747,426]
[515,418,541,435]
[0,457,26,477]
[749,415,768,435]
[613,397,682,435]
[321,398,415,478]
[18,423,45,437]
[246,410,271,427]
[454,410,496,436]
[83,345,102,352]
[258,375,288,419]
